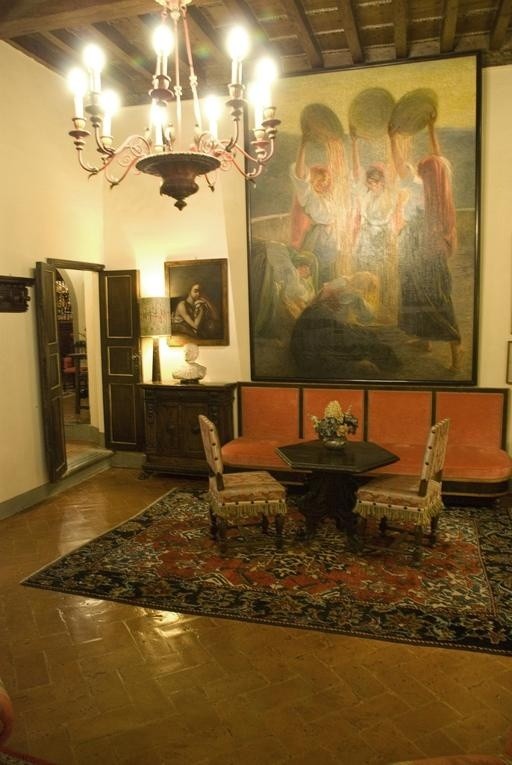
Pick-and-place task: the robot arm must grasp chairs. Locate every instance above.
[357,417,449,566]
[60,356,81,391]
[198,415,286,557]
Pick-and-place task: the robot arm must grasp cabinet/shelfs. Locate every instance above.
[139,382,235,481]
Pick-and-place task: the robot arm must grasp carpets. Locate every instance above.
[19,487,512,654]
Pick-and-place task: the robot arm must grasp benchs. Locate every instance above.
[220,381,512,508]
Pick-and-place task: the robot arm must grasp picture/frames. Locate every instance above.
[243,52,481,386]
[164,258,229,347]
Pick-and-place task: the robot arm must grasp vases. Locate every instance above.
[322,438,348,450]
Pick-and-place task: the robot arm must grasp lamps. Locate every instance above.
[139,297,172,383]
[68,0,282,211]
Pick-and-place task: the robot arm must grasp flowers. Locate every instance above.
[307,400,358,437]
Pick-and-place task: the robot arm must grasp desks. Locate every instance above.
[70,353,88,414]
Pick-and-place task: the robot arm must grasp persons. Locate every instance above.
[172,343,207,379]
[173,280,220,339]
